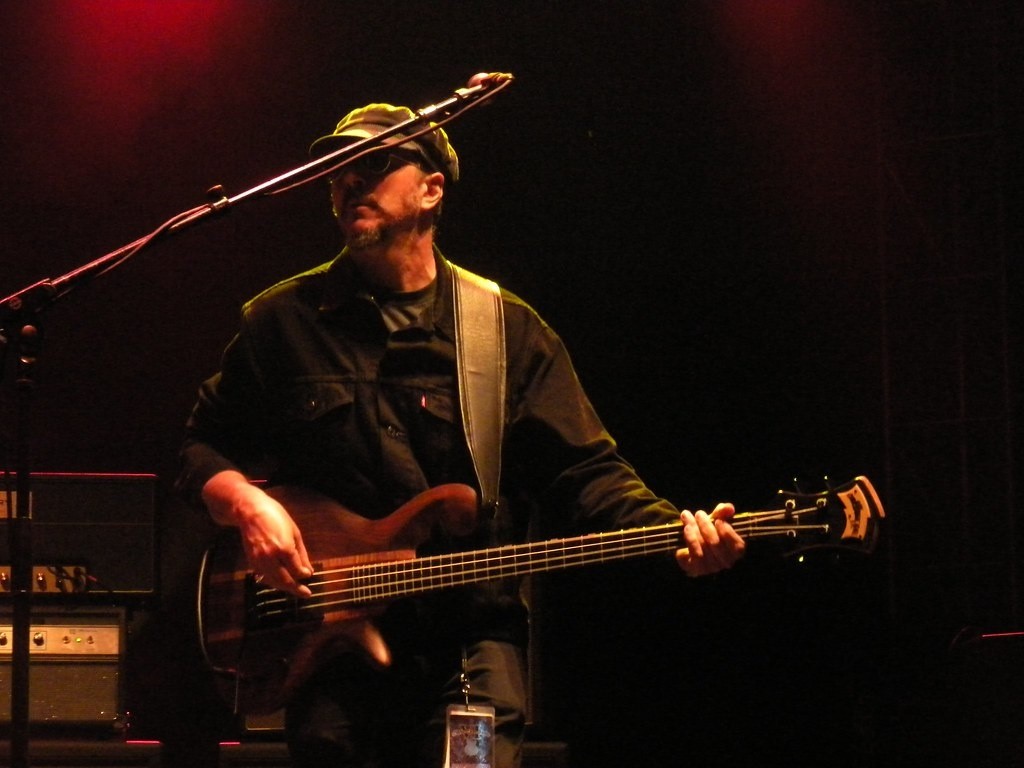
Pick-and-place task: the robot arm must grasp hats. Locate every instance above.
[308,102,460,186]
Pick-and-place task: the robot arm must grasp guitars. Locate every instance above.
[193,473,889,720]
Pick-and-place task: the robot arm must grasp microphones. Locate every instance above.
[467,72,513,90]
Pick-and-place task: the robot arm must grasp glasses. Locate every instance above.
[322,144,427,185]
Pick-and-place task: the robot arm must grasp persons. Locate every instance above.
[154,100,746,766]
